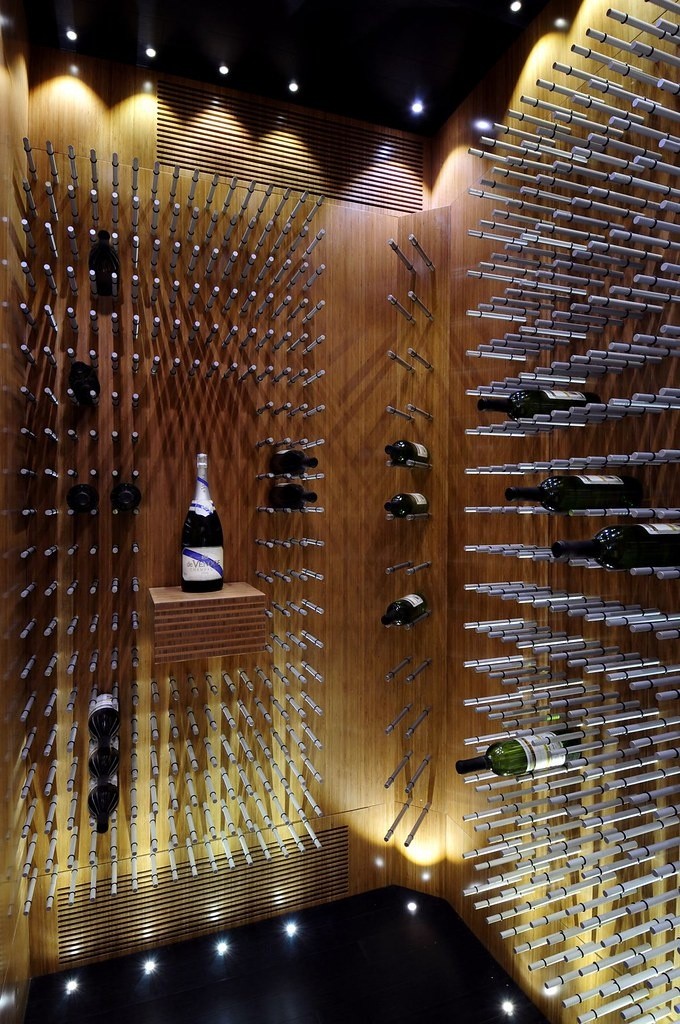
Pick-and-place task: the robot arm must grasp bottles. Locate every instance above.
[90,690,119,836]
[182,453,224,594]
[64,481,99,513]
[385,440,429,467]
[455,727,580,779]
[271,449,317,510]
[381,594,426,626]
[552,524,680,573]
[505,475,643,512]
[89,230,119,300]
[383,492,427,518]
[67,363,100,403]
[112,484,140,512]
[477,389,601,420]
